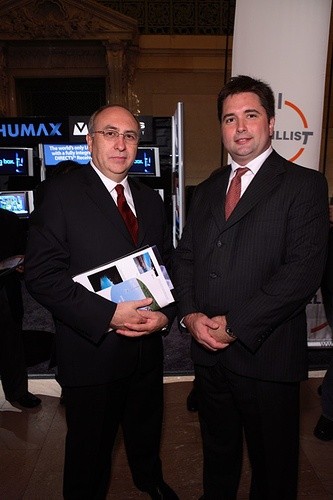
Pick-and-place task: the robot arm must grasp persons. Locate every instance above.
[171,75,330,500]
[25,102,180,500]
[0,208,42,408]
[312,197,333,444]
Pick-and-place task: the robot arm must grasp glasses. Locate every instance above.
[92,130,139,145]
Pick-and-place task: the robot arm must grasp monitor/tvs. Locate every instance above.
[0,147,34,177]
[0,190,35,219]
[127,147,160,176]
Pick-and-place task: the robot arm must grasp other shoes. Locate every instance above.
[315,415,333,441]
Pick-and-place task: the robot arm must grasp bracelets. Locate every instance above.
[225,323,238,339]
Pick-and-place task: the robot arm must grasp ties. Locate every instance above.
[224,168,251,222]
[114,184,138,247]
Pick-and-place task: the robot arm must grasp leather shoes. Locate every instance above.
[147,481,180,500]
[187,390,198,412]
[16,391,42,408]
